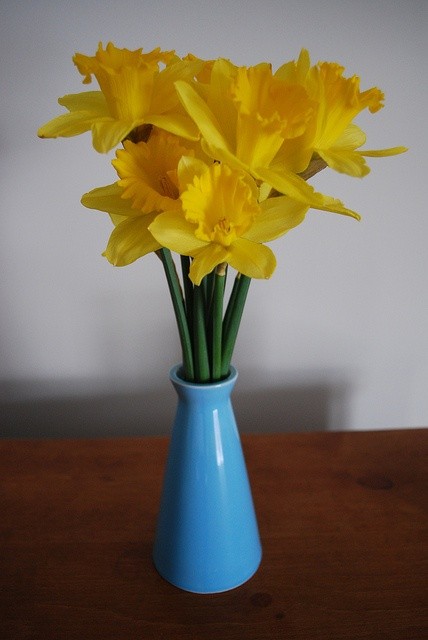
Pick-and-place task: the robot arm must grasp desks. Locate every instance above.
[0,429,428,640]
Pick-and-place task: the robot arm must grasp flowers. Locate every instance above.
[32,43,409,380]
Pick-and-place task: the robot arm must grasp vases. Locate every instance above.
[151,367,262,597]
